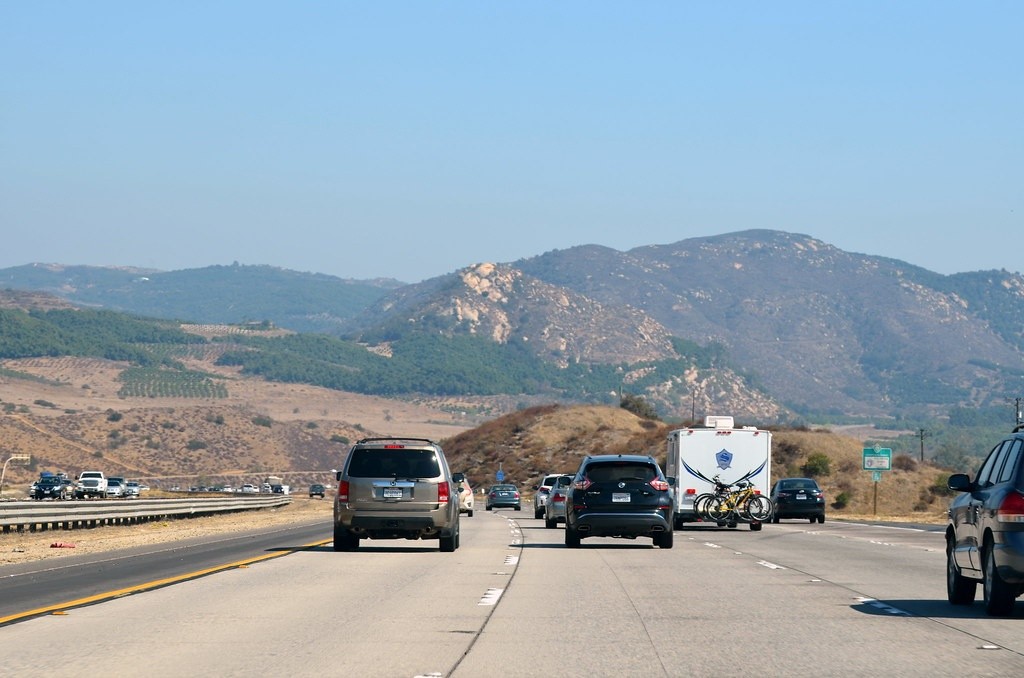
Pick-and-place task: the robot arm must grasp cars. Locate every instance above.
[771,478,825,523]
[486,484,521,511]
[190,475,325,498]
[30,471,149,500]
[532,455,675,548]
[945,425,1024,617]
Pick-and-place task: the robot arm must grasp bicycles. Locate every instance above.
[693,474,773,521]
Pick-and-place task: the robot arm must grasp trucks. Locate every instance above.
[666,416,772,530]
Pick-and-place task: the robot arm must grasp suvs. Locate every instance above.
[333,438,473,552]
